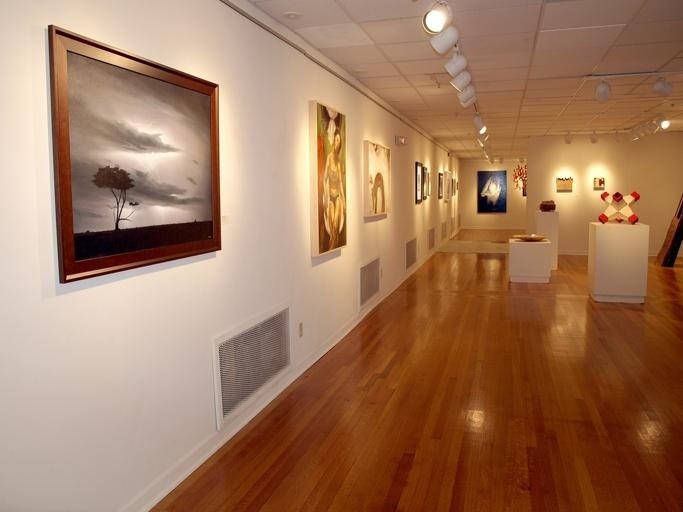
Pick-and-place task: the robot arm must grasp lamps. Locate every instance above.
[651,76,672,96]
[594,79,612,103]
[628,116,670,142]
[564,131,574,145]
[589,130,601,144]
[472,116,495,165]
[422,1,479,109]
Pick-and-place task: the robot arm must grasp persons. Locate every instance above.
[321,131,344,251]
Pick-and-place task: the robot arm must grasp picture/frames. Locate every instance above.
[412,163,458,203]
[48,24,221,281]
[311,100,348,256]
[363,141,391,222]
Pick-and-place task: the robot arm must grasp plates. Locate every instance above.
[512,233,545,241]
